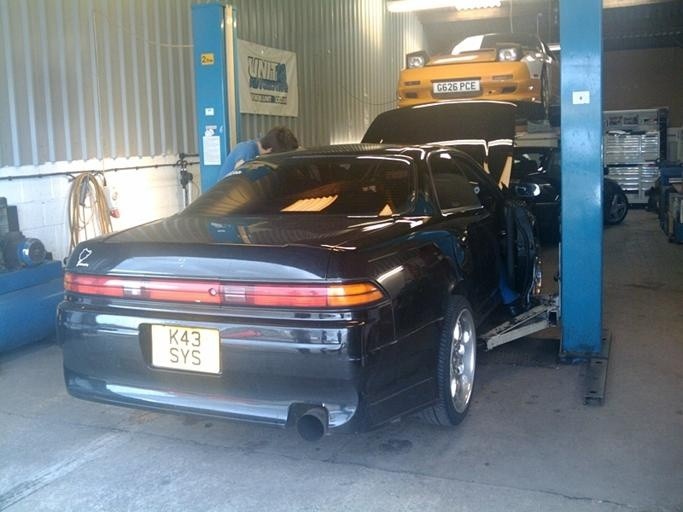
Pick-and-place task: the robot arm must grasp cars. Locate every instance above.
[55,99,544,445]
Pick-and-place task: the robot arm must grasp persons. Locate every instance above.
[207,127,299,244]
